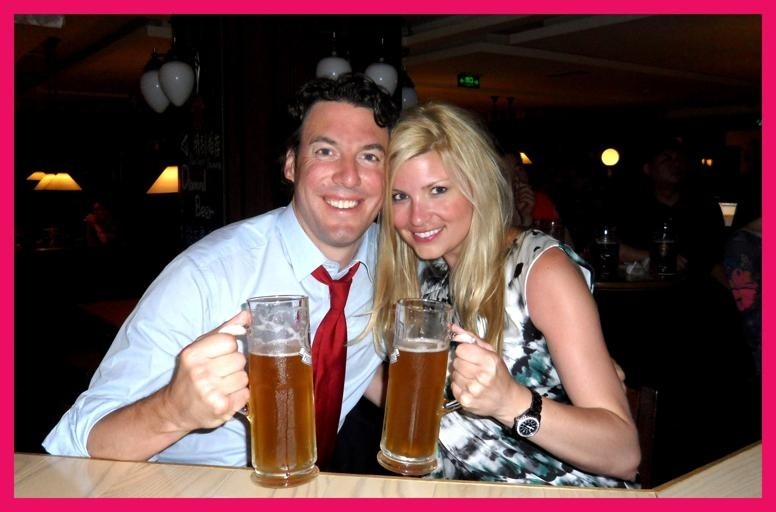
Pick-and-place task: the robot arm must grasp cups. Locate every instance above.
[219,294,320,489]
[377,298,479,474]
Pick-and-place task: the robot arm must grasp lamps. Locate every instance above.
[25,165,83,192]
[140,35,198,113]
[146,165,180,196]
[599,147,621,179]
[315,29,420,113]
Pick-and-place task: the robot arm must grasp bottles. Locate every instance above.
[595,221,619,282]
[655,217,679,282]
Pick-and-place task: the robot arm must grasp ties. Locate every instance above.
[312,262,359,467]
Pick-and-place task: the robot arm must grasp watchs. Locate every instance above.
[510,391,540,439]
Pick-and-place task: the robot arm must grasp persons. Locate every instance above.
[348,102,642,488]
[84,198,118,241]
[40,72,410,461]
[503,119,760,374]
